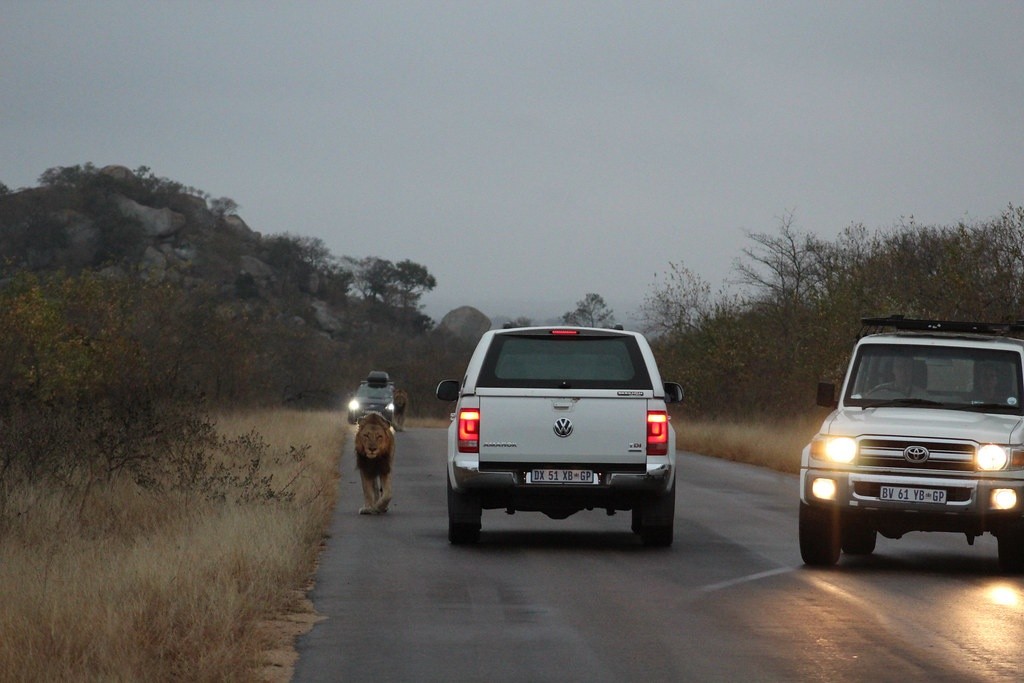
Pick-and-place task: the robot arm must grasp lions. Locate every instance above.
[354,410,396,514]
[394,388,407,433]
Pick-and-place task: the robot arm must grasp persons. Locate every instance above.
[863,354,932,401]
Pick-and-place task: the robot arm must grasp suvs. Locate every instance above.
[347,371,396,425]
[798,314,1024,576]
[436,322,684,547]
